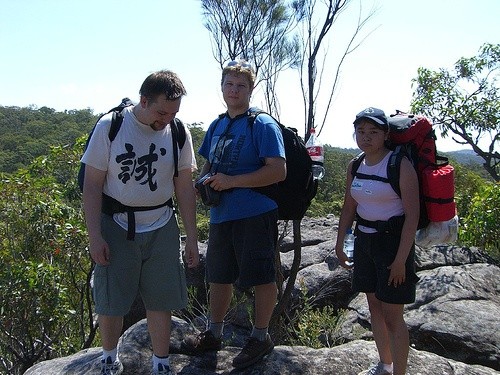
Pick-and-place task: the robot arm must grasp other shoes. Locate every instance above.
[358,361,392,375]
[101,356,123,375]
[151,362,172,375]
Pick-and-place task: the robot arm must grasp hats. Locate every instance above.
[353,107,388,127]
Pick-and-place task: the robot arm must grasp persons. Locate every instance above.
[81,70,200,375]
[182,59,287,367]
[336,107,420,375]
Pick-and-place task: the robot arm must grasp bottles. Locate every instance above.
[304,128,324,180]
[343,228,355,266]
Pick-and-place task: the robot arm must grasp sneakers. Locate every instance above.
[196,331,221,367]
[232,333,274,368]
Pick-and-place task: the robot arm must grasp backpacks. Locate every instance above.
[215,111,318,221]
[77,97,186,215]
[351,112,458,247]
